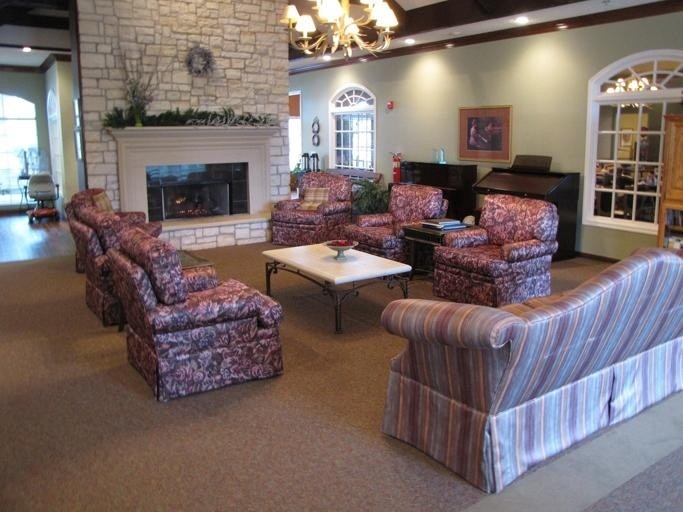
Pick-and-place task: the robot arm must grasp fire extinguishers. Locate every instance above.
[390,151,402,184]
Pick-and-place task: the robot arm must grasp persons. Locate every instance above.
[595,160,657,213]
[468,120,483,149]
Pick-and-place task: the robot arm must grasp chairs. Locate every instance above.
[593,164,657,224]
[107,226,284,402]
[272,171,352,246]
[0,173,60,224]
[64,188,161,274]
[433,194,559,308]
[344,184,449,264]
[71,208,216,326]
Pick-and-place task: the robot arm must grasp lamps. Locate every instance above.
[604,77,656,109]
[279,0,399,60]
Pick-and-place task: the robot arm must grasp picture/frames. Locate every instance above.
[620,127,633,146]
[457,104,511,163]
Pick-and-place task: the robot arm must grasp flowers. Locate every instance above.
[124,80,152,111]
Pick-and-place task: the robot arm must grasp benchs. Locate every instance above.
[324,169,382,186]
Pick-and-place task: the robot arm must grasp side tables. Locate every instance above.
[401,219,471,280]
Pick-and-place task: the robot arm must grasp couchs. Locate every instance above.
[379,246,682,494]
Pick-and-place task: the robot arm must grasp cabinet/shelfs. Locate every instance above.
[402,160,477,219]
[656,114,682,250]
[472,167,579,261]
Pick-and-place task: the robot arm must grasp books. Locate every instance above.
[418,217,467,232]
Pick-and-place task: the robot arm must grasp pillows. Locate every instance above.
[297,201,319,210]
[303,186,331,202]
[93,190,113,214]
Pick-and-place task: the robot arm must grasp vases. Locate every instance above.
[133,109,143,127]
